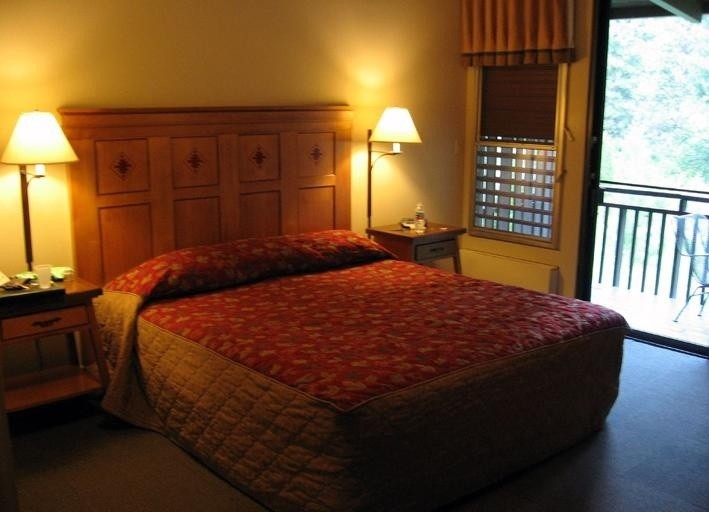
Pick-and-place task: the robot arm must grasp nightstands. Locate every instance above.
[366,220,467,274]
[1,274,110,414]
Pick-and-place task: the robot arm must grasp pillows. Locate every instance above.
[105,230,393,304]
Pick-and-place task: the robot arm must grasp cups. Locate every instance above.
[33,264,53,290]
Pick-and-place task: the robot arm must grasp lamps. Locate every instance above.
[1,112,80,188]
[367,106,422,170]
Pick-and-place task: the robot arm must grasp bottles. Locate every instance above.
[414,200,425,234]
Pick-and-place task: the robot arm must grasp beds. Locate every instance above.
[58,106,627,512]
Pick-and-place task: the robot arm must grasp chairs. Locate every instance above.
[670,213,709,322]
